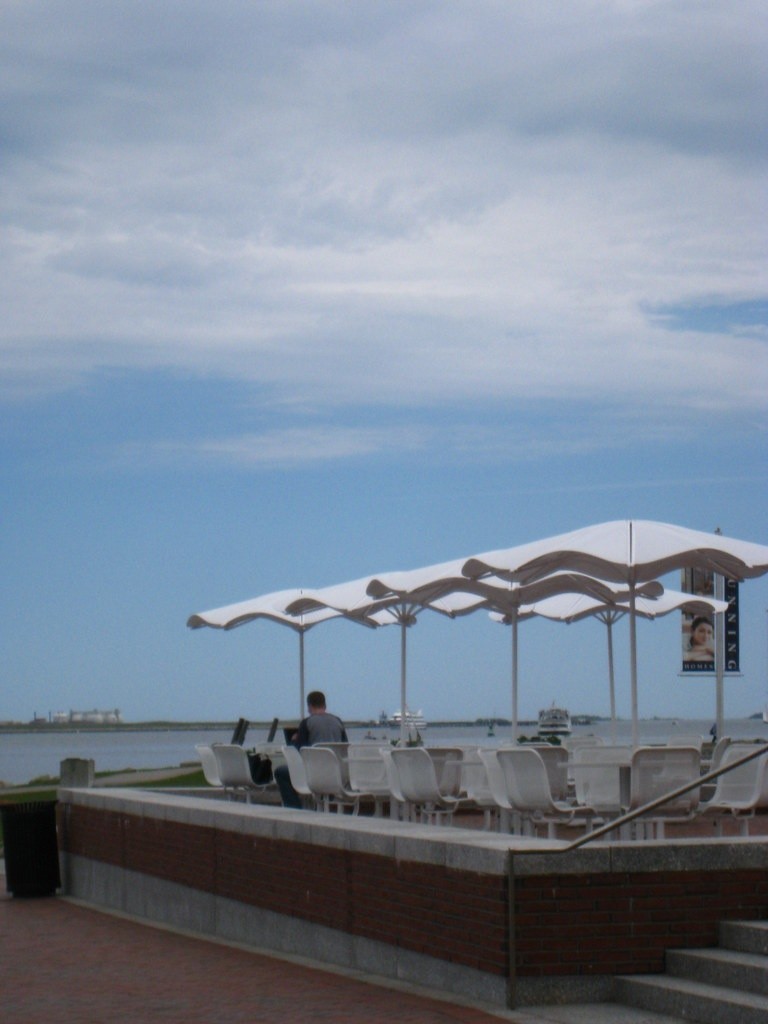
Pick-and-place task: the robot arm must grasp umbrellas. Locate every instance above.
[285,572,488,748]
[488,589,730,744]
[366,549,663,744]
[185,587,396,724]
[462,520,768,757]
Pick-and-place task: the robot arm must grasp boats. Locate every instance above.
[537,701,575,736]
[387,709,428,731]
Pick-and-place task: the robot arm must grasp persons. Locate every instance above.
[275,692,348,810]
[681,618,715,662]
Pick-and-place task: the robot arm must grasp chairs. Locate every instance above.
[194,735,768,841]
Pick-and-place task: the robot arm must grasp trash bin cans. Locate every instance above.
[0,798,62,899]
